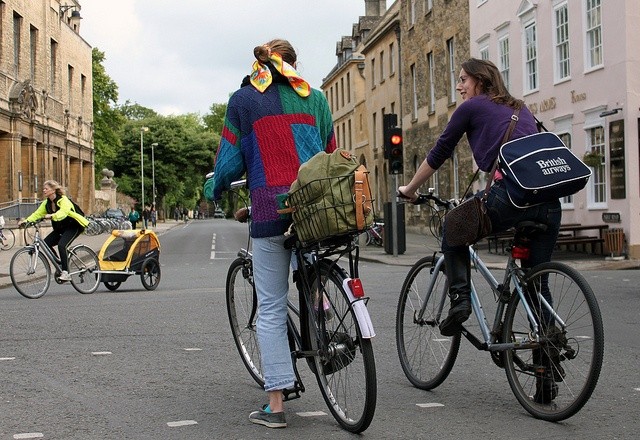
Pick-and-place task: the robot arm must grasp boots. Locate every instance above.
[532,344,559,403]
[439,246,472,334]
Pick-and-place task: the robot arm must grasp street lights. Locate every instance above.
[141,126,148,231]
[151,143,159,211]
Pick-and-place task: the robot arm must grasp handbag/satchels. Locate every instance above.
[495,132,593,212]
[288,149,376,247]
[136,217,139,223]
[148,216,152,221]
[442,196,492,251]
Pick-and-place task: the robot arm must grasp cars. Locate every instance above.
[214,210,224,218]
[104,207,125,217]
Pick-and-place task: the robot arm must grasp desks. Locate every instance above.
[560,223,609,232]
[496,229,516,236]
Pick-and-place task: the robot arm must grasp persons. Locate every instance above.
[174,207,188,222]
[202,38,339,430]
[151,208,157,227]
[128,206,140,229]
[398,57,563,404]
[143,206,150,229]
[18,179,89,282]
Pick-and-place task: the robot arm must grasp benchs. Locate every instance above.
[487,237,515,256]
[553,233,606,256]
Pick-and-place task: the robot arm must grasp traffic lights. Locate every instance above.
[389,129,404,175]
[383,114,397,159]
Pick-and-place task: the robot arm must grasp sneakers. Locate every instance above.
[57,271,73,282]
[248,403,288,428]
[314,305,334,321]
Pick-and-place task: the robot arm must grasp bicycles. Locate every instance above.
[9,217,101,299]
[205,172,376,433]
[353,216,385,247]
[83,214,132,236]
[395,188,604,422]
[0,216,15,251]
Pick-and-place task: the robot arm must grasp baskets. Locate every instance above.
[282,168,376,245]
